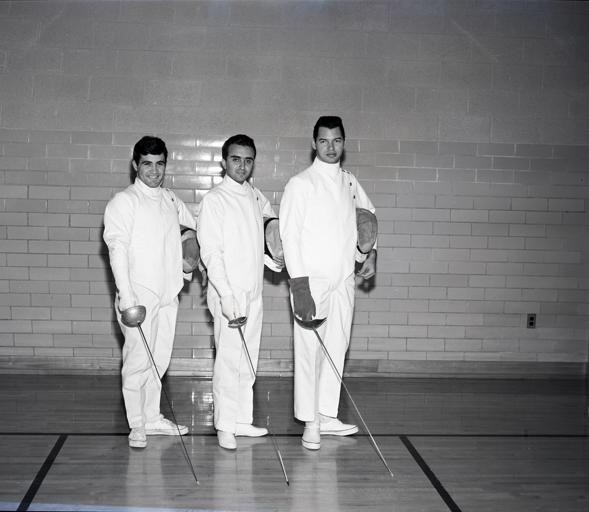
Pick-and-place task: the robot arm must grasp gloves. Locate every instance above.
[117,289,139,312]
[220,295,241,320]
[289,276,317,321]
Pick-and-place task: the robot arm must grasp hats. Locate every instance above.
[355,208,378,263]
[181,226,200,282]
[263,216,286,273]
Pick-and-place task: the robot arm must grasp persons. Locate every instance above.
[210,435,269,512]
[288,432,359,511]
[278,114,378,445]
[124,434,175,511]
[197,131,280,455]
[102,135,198,449]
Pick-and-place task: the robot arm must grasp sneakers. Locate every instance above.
[128,426,147,448]
[237,425,267,437]
[301,426,321,450]
[319,417,358,435]
[216,429,237,449]
[147,419,189,435]
